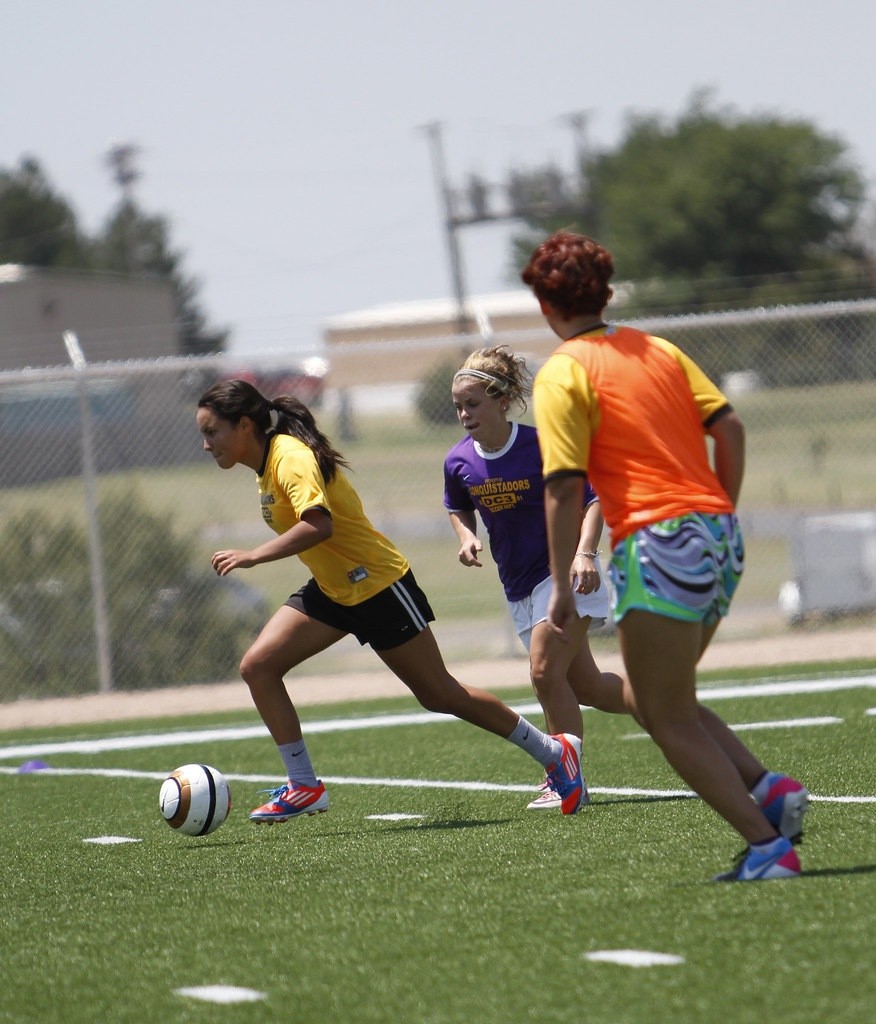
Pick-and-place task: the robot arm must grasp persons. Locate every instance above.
[517,228,807,881]
[198,376,583,826]
[442,344,629,810]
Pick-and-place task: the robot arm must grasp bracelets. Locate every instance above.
[572,551,602,560]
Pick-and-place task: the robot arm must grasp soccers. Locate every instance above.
[158,763,233,836]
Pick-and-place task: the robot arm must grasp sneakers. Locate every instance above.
[714,838,803,880]
[541,733,588,815]
[528,790,565,810]
[251,780,329,826]
[758,774,810,848]
